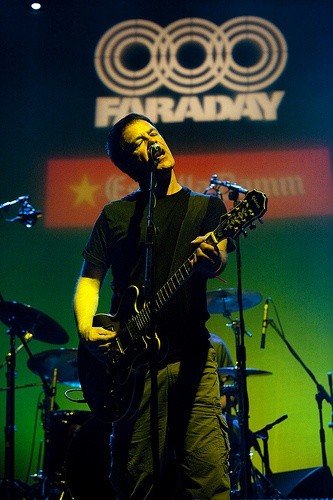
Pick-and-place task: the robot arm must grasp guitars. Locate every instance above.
[77,189,268,422]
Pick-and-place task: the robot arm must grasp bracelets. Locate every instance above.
[216,252,223,272]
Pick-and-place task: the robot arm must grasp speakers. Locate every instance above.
[231,467,333,500]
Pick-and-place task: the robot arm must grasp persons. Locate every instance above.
[71,113,238,500]
[208,333,240,411]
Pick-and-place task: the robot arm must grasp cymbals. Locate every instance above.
[1,300,69,345]
[207,290,263,315]
[27,349,81,388]
[217,367,273,378]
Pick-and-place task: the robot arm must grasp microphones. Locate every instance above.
[18,200,43,228]
[259,300,268,348]
[148,143,162,160]
